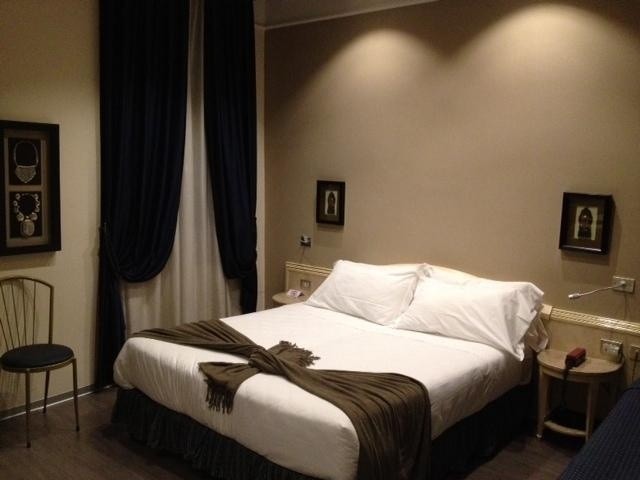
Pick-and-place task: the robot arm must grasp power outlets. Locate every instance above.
[629,344,640,360]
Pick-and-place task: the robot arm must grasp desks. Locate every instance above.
[534,347,625,445]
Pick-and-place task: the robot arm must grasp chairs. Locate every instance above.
[0,276,81,448]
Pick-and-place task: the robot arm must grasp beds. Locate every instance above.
[113,300,537,480]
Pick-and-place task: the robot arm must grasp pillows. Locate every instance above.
[305,260,543,361]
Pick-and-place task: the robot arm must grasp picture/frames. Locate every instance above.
[314,179,344,225]
[558,190,614,256]
